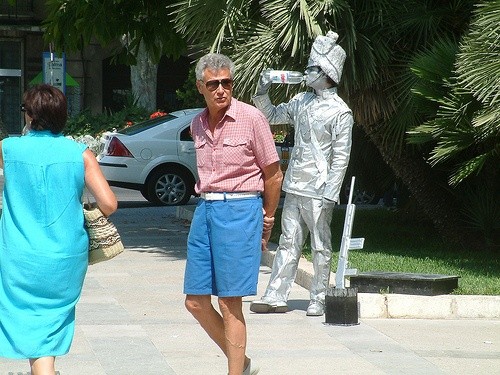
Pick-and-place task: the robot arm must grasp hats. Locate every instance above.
[306,30,346,85]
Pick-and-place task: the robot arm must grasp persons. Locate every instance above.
[0,82,118,375]
[184,53,283,374]
[250,29,354,317]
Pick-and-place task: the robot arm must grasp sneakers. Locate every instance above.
[249,301,289,313]
[306,299,325,315]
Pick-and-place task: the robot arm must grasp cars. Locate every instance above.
[95,108,294,206]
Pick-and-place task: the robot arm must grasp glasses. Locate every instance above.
[20,104,26,112]
[201,78,234,92]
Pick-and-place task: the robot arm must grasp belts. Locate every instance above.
[200,191,261,201]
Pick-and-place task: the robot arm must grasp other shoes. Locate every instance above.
[243,358,260,375]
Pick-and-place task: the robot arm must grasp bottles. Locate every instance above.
[265,71,306,85]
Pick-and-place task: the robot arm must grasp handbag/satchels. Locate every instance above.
[83,201,125,266]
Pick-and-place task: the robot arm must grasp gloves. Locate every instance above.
[318,198,335,210]
[255,68,275,95]
